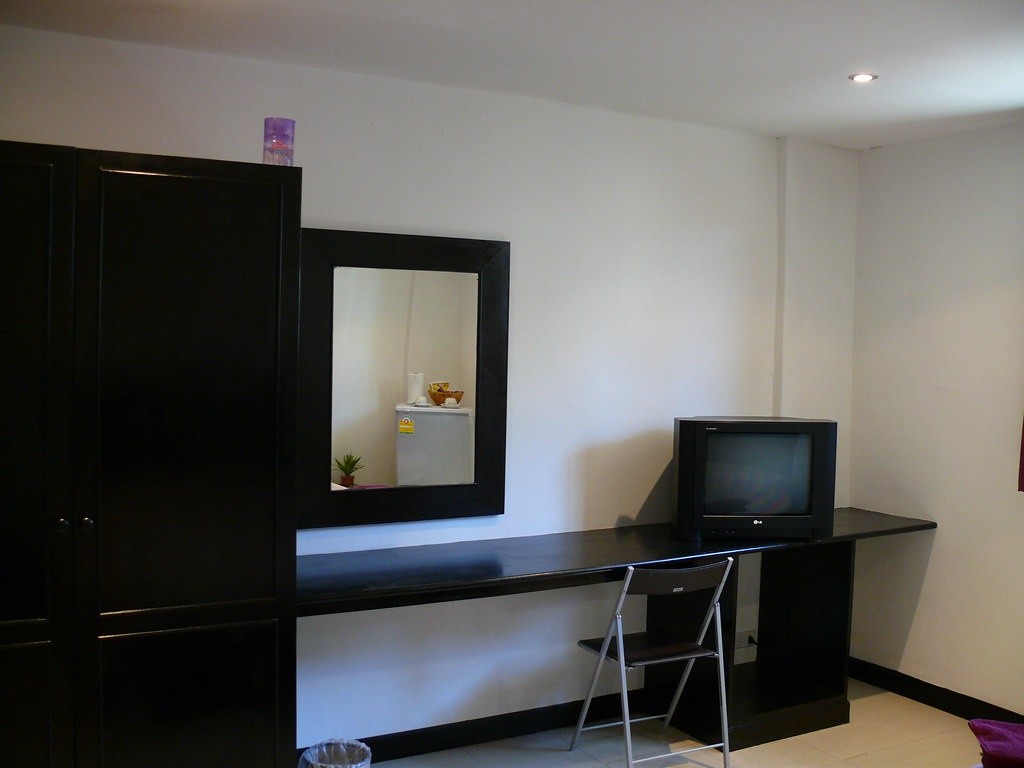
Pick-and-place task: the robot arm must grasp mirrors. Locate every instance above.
[297,228,511,529]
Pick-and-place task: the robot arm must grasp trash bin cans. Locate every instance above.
[302,742,371,768]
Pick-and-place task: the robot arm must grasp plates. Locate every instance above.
[414,404,432,407]
[441,405,459,408]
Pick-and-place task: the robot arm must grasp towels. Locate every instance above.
[967,719,1024,768]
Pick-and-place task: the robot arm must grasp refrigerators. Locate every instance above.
[396,403,473,485]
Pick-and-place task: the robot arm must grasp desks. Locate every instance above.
[297,508,937,752]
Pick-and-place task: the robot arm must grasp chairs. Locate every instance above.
[569,557,733,768]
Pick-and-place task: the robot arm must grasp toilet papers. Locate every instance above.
[407,372,424,402]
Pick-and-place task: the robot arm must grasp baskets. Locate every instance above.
[428,382,464,407]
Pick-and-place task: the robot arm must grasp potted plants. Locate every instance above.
[332,453,365,486]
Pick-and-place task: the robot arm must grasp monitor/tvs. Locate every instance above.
[672,417,837,545]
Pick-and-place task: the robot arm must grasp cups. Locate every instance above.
[262,116,296,166]
[445,398,457,406]
[416,396,428,404]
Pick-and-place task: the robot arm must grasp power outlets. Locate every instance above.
[735,630,756,648]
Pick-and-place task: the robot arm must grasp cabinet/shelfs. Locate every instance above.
[0,139,302,768]
